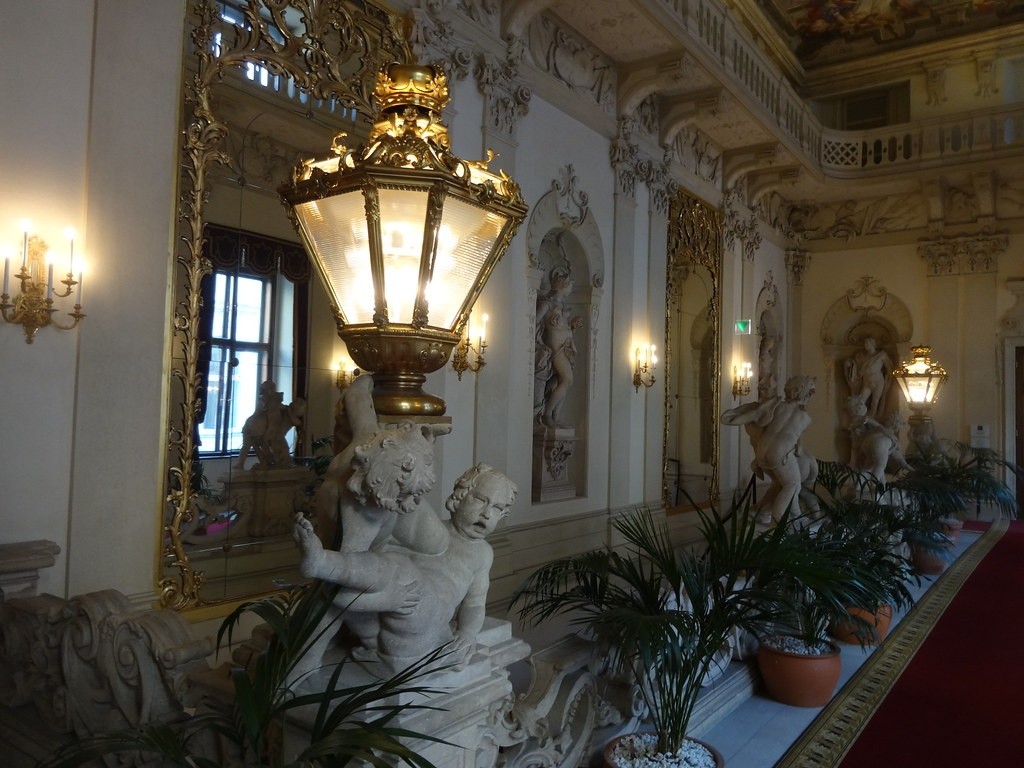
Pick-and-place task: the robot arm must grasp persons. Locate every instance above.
[229,380,308,470]
[536,264,584,429]
[720,374,825,528]
[840,394,917,506]
[843,336,895,420]
[265,374,522,708]
[758,336,778,391]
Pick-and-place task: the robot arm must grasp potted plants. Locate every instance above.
[511,441,1024,768]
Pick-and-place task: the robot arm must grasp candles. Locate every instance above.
[3,257,9,294]
[463,312,490,355]
[74,272,83,308]
[23,229,29,267]
[48,261,53,299]
[635,342,658,377]
[68,234,74,279]
[735,362,754,381]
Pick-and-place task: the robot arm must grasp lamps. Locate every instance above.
[892,346,947,413]
[289,60,529,411]
